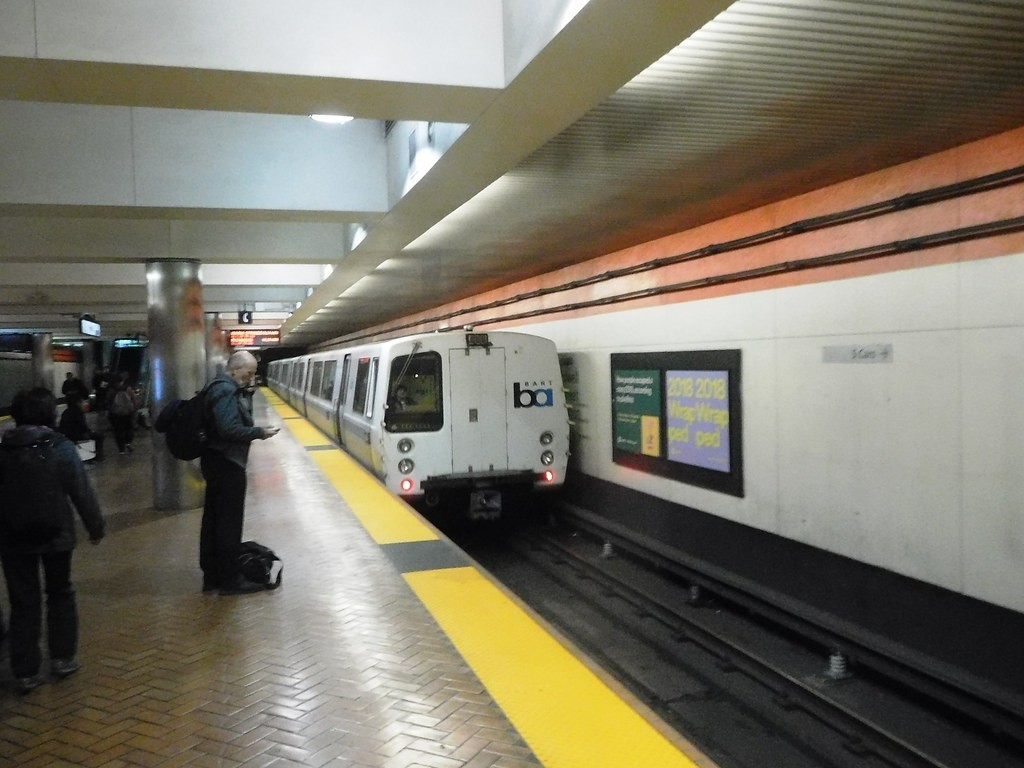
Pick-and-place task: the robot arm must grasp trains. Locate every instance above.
[268,324,573,526]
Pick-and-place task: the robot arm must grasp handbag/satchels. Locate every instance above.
[233,542,284,589]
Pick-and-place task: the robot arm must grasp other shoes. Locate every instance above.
[219,577,264,595]
[15,656,52,688]
[203,572,222,591]
[52,656,82,673]
[125,441,131,446]
[119,452,125,454]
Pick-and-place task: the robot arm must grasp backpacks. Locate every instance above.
[155,380,228,461]
[110,384,132,417]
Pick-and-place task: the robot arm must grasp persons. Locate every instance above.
[199,350,282,594]
[388,383,417,413]
[0,389,106,690]
[59,365,149,459]
[325,380,333,398]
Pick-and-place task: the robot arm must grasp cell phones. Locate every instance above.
[272,428,280,431]
